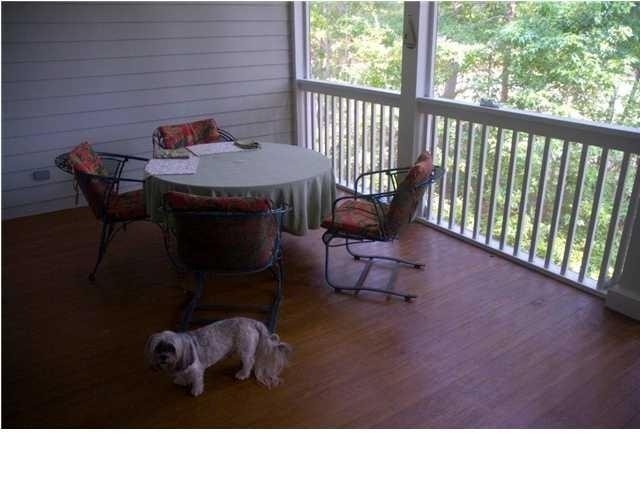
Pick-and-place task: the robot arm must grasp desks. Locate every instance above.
[144,141,337,236]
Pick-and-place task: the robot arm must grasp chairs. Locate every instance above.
[54,140,178,282]
[318,150,445,304]
[152,118,240,159]
[156,191,294,335]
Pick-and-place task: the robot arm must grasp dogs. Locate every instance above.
[147,316,291,398]
